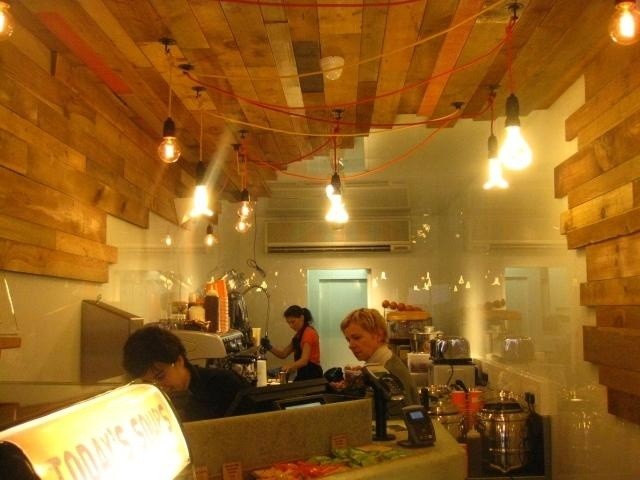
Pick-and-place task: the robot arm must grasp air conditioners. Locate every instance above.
[264,216,413,256]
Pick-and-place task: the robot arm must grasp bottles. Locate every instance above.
[205,285,220,334]
[466,423,484,479]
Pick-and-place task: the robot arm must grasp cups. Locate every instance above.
[252,327,261,347]
[256,360,267,386]
[451,388,483,433]
[215,280,229,333]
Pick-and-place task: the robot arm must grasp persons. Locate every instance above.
[260,305,323,383]
[122,325,258,422]
[334,309,420,418]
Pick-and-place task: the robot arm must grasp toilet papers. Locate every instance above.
[256,360,268,388]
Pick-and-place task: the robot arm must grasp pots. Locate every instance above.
[428,336,472,363]
[502,336,536,364]
